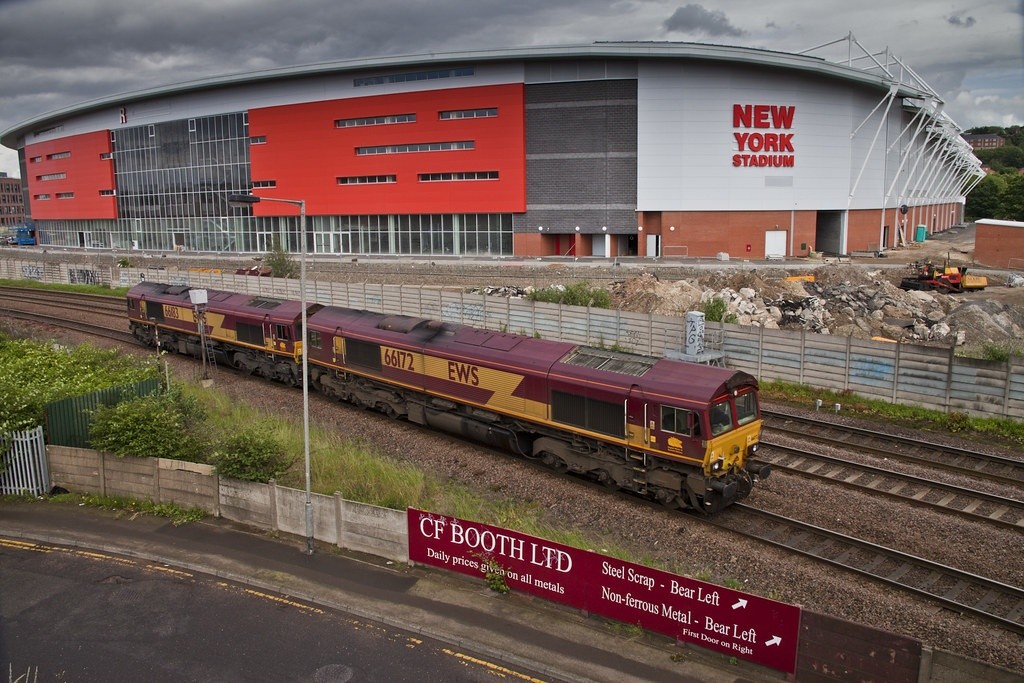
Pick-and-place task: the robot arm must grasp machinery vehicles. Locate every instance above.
[899,252,988,295]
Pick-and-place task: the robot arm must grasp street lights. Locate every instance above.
[227,191,319,557]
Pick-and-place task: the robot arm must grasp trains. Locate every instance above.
[126,279,772,520]
